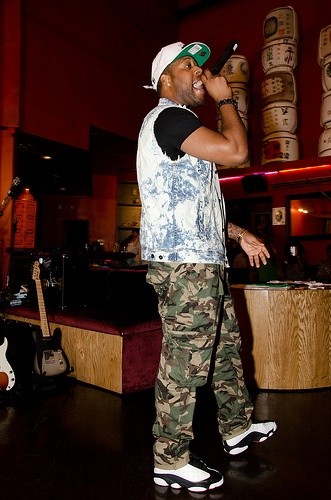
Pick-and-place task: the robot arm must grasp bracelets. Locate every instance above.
[236,229,248,243]
[217,98,238,110]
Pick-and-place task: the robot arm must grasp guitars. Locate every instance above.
[30,260,69,378]
[0,337,16,393]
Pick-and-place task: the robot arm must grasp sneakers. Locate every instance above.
[153,454,224,492]
[222,422,277,455]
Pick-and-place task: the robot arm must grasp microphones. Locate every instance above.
[209,39,239,76]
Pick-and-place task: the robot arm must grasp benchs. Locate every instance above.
[0,307,162,394]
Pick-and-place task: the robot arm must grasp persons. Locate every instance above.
[248,222,331,284]
[135,42,277,493]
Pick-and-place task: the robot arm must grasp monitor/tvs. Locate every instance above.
[12,132,94,195]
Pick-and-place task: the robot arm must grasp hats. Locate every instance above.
[143,42,211,92]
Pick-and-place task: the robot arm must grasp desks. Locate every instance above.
[230,284,331,390]
